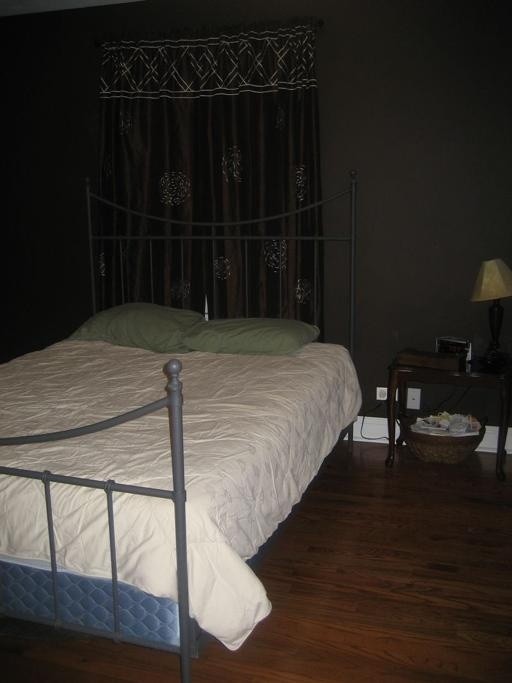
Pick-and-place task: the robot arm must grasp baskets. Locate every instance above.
[403,408,488,465]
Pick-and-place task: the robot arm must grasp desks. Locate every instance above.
[383,343,510,482]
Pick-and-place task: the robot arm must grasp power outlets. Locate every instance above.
[376,385,390,402]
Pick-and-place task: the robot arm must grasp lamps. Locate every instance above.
[471,256,512,373]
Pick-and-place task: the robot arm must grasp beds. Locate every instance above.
[0,171,358,682]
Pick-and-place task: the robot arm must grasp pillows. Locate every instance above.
[179,314,322,354]
[70,298,208,354]
